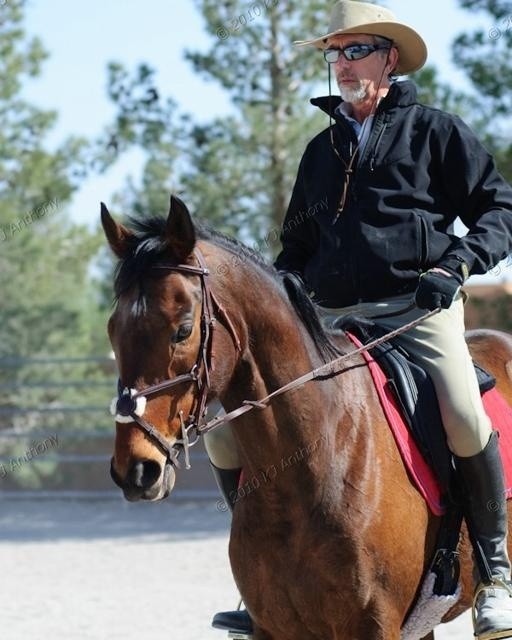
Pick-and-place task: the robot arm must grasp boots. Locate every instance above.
[442,427,510,640]
[208,455,257,634]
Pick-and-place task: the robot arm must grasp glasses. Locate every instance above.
[322,41,390,65]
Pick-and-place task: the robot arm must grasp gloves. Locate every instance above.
[413,255,471,314]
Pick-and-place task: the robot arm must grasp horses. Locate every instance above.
[99,194,511,640]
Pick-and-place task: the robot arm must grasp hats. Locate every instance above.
[290,1,429,78]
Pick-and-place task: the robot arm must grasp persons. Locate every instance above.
[204,0,511,640]
